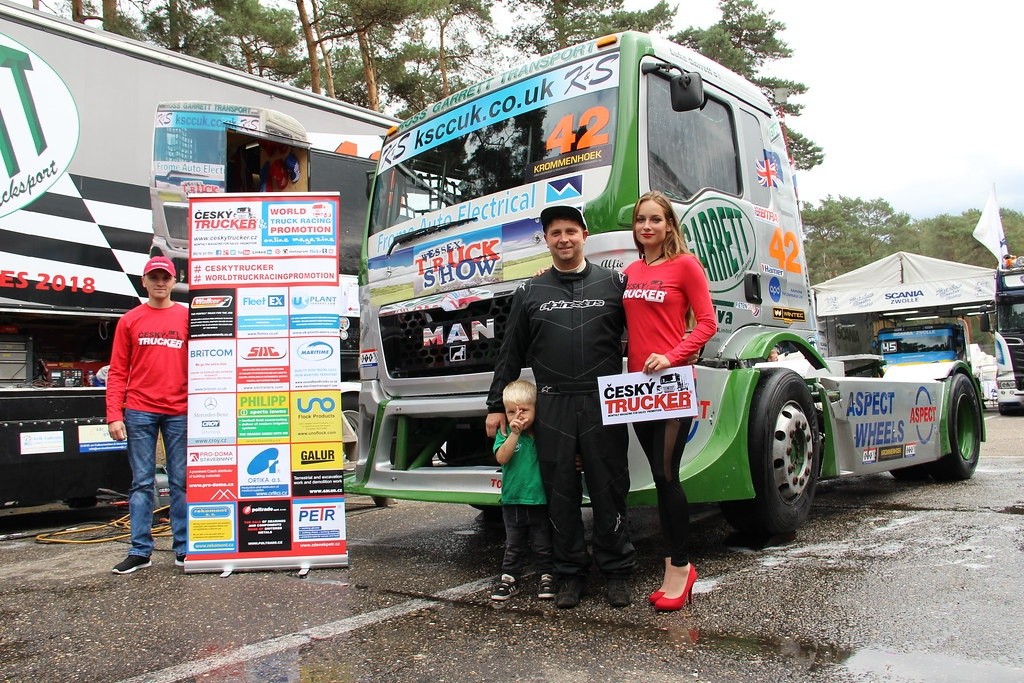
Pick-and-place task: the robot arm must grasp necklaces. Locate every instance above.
[643,255,665,266]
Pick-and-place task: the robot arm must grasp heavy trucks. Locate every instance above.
[343,28,986,536]
[871,322,970,378]
[0,0,406,519]
[979,254,1024,416]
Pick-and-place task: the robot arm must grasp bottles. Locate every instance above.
[87,371,96,385]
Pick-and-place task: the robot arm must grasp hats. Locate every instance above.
[542,206,586,231]
[144,256,177,278]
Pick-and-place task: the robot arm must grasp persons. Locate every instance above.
[618,191,717,610]
[486,379,561,600]
[484,202,640,610]
[104,257,190,575]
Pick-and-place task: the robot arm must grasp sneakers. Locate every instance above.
[113,553,184,575]
[557,577,631,608]
[492,573,555,601]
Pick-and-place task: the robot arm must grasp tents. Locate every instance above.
[809,249,998,357]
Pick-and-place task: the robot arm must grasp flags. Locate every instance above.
[972,191,1010,268]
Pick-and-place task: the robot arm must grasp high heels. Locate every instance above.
[648,563,698,611]
